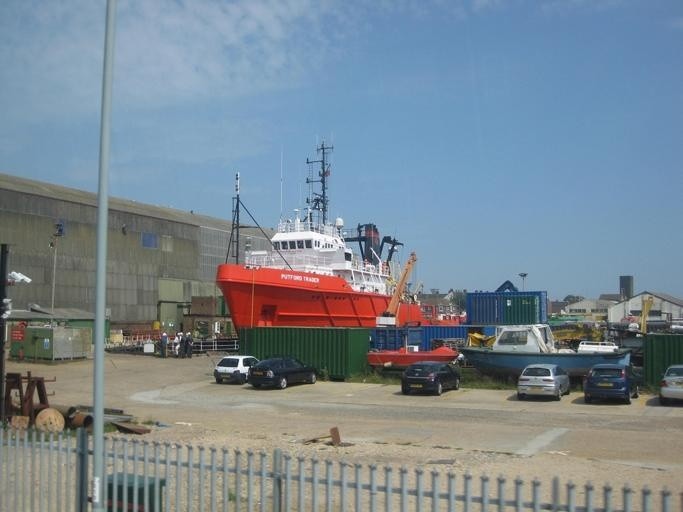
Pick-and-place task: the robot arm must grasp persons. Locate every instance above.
[161,332,193,358]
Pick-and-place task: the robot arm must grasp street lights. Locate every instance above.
[517,272,528,292]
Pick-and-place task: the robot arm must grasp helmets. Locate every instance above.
[162,332,191,336]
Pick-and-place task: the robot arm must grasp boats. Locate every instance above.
[366,346,459,370]
[457,323,632,384]
[215,140,467,342]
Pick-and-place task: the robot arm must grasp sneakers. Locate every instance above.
[173,354,192,359]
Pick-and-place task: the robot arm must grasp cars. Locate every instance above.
[659,364,683,405]
[582,363,635,404]
[213,355,260,385]
[401,360,460,396]
[247,355,317,389]
[516,363,569,401]
